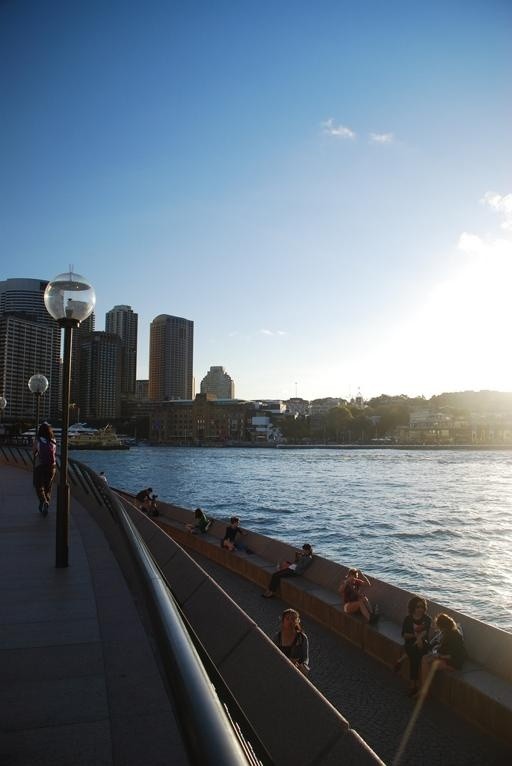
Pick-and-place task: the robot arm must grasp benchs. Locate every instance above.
[146,508,512,756]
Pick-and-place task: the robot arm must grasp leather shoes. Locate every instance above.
[261,594,273,599]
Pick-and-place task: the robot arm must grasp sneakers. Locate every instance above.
[38,500,50,516]
[391,662,401,673]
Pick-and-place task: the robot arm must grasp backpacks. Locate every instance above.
[37,438,56,469]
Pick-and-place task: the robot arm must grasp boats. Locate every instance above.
[14,422,136,451]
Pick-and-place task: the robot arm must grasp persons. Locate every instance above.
[98,471,108,485]
[220,516,249,551]
[135,486,153,507]
[273,607,310,675]
[410,612,467,699]
[261,543,313,597]
[32,421,57,517]
[392,595,433,693]
[339,567,381,625]
[185,508,209,535]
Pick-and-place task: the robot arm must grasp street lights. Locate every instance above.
[0,374,49,486]
[43,265,96,567]
